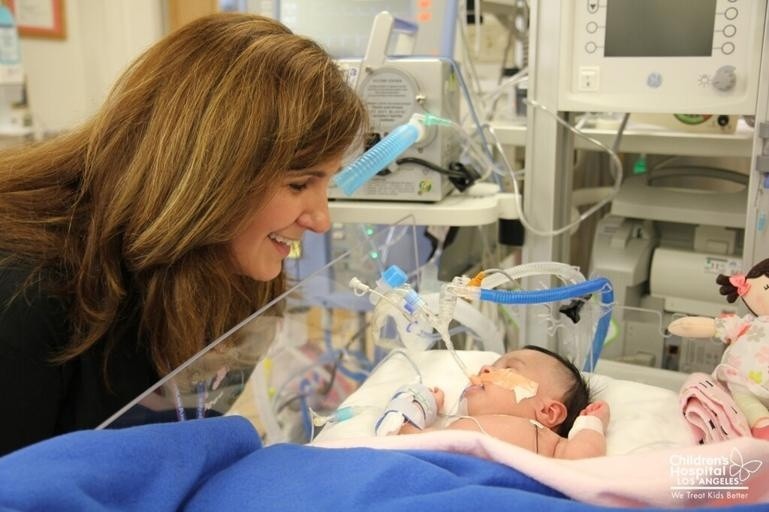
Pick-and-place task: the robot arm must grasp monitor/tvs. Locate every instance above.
[558,0,767,116]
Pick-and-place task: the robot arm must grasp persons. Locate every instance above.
[395,343,609,459]
[665,259,769,445]
[0,11,368,461]
